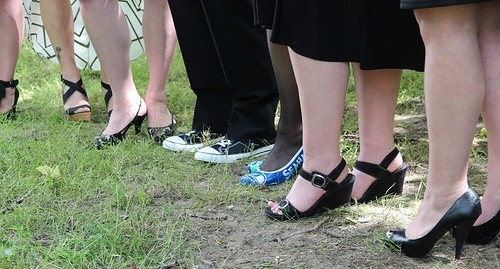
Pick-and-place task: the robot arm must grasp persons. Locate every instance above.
[240,1,304,186]
[163,0,280,164]
[1,1,177,150]
[265,0,426,221]
[382,1,499,260]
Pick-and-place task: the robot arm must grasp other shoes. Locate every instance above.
[240,145,304,186]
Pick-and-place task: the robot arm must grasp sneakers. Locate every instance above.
[195,138,274,163]
[163,131,225,152]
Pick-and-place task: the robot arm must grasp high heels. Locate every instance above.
[88,98,148,150]
[0,79,19,120]
[386,188,482,260]
[61,74,91,121]
[148,108,176,146]
[101,81,113,117]
[449,209,500,246]
[265,157,356,222]
[350,147,407,206]
